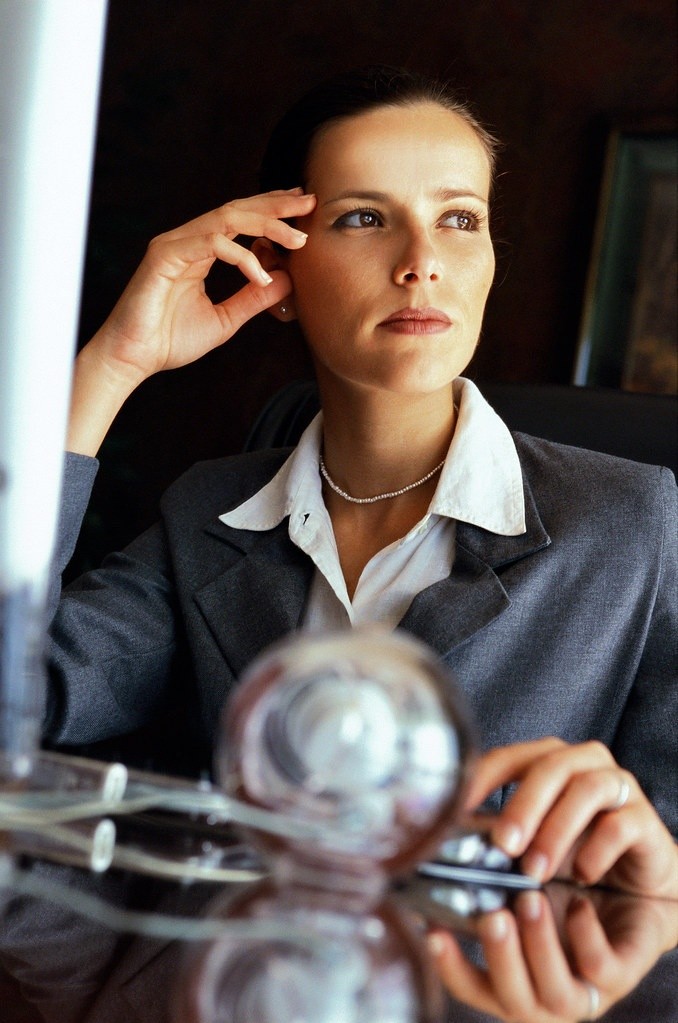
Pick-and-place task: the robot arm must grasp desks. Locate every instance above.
[0,752,678,1023]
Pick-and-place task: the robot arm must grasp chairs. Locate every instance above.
[244,379,678,490]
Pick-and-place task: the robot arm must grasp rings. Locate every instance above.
[583,981,599,1021]
[607,768,630,811]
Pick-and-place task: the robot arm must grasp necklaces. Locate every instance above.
[320,402,461,505]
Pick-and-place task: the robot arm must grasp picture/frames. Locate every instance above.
[566,113,678,395]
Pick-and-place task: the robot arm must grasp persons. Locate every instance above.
[42,64,678,893]
[0,850,678,1023]
